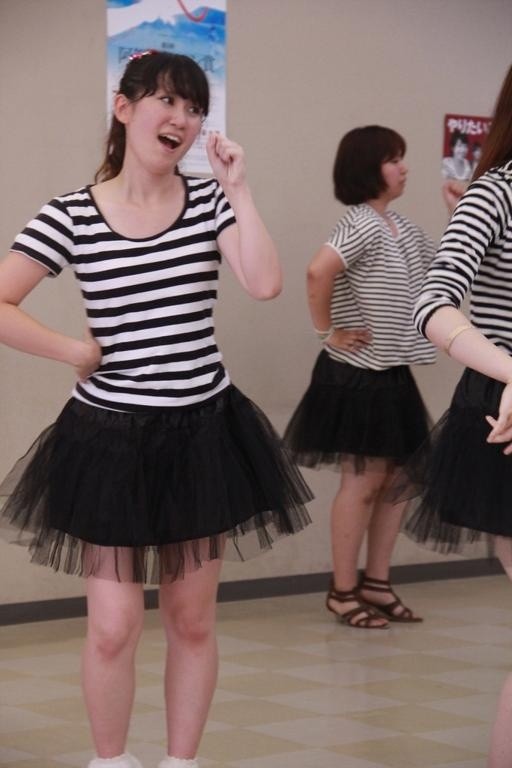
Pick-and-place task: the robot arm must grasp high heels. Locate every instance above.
[325,574,426,629]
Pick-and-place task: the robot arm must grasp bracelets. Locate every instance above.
[445,323,477,358]
[312,326,334,343]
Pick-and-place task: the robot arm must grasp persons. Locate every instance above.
[410,63,510,586]
[0,48,312,765]
[470,143,483,177]
[440,132,470,177]
[279,128,468,632]
